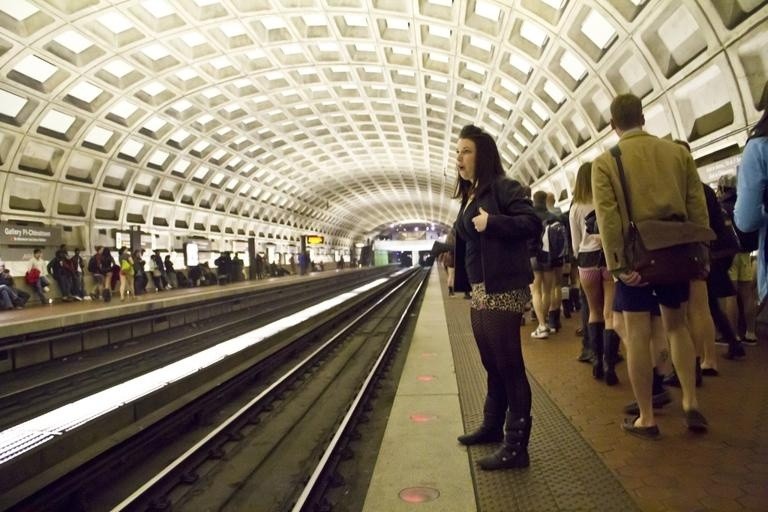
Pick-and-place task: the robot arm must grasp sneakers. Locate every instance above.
[530,309,538,321]
[623,388,672,414]
[621,415,663,441]
[652,329,758,389]
[576,348,594,361]
[685,408,708,434]
[576,328,584,336]
[531,325,550,339]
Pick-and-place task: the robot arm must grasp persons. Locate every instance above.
[588,93,714,441]
[733,80,768,308]
[449,123,544,471]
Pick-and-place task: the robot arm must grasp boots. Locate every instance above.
[569,287,583,312]
[457,397,508,445]
[562,298,572,318]
[586,320,606,380]
[548,308,562,334]
[476,409,533,471]
[601,327,620,386]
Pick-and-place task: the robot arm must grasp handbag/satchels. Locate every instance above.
[25,268,41,284]
[120,259,132,273]
[625,218,718,285]
